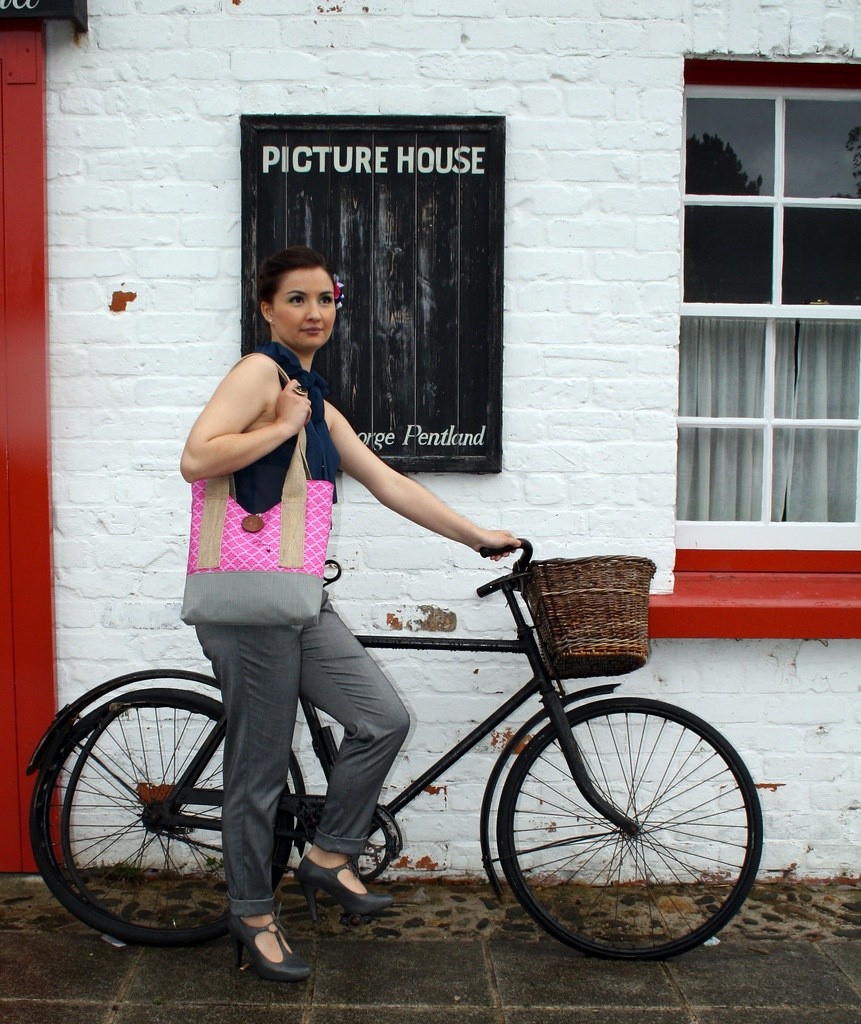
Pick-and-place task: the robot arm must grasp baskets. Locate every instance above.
[523,555,656,678]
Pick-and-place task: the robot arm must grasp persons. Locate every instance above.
[173,245,520,983]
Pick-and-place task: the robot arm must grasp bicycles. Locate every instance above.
[23,535,766,962]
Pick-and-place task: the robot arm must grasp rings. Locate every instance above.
[292,386,309,398]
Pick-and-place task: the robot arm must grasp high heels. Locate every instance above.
[298,855,396,922]
[229,912,311,983]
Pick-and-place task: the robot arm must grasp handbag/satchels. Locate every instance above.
[181,354,335,623]
